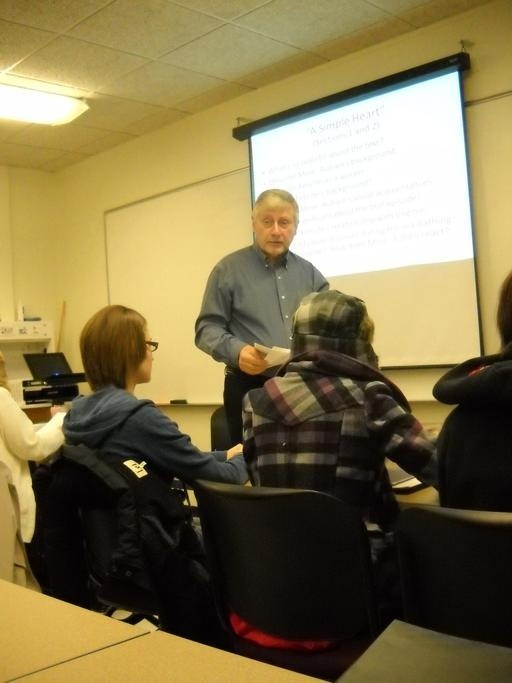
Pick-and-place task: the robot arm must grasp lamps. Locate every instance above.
[1,79,92,126]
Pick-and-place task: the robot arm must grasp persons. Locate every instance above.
[61,304,249,616]
[430,269,512,516]
[194,186,329,450]
[0,354,68,594]
[239,288,442,642]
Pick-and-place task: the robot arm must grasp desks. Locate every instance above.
[6,625,326,681]
[0,575,151,682]
[333,614,512,681]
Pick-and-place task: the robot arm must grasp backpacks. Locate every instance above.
[19,446,177,622]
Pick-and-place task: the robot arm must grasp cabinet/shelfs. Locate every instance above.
[21,403,58,426]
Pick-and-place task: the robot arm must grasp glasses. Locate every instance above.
[142,339,158,351]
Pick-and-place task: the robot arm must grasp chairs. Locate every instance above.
[16,446,213,636]
[188,468,386,675]
[385,496,512,652]
[0,460,37,591]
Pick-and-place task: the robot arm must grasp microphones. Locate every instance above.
[41,348,47,385]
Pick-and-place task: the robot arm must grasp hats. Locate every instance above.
[288,289,380,371]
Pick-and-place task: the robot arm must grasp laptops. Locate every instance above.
[23,352,76,386]
